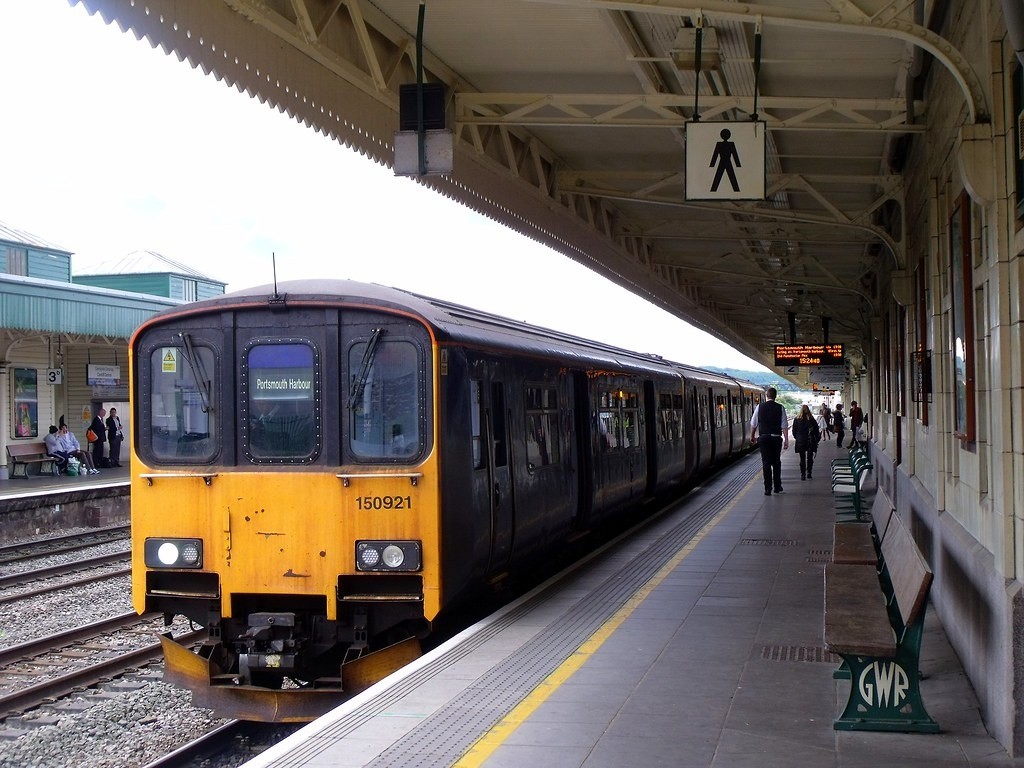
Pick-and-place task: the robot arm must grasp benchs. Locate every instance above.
[6,442,78,480]
[823,487,942,734]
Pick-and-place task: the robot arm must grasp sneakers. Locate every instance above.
[93,468,100,474]
[87,469,96,475]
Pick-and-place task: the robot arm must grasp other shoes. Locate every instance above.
[113,463,122,467]
[807,474,812,478]
[55,466,62,476]
[94,463,101,468]
[801,475,806,480]
[764,489,771,495]
[774,486,783,493]
[846,446,852,449]
[838,447,845,449]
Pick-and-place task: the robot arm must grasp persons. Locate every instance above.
[106,408,124,467]
[88,409,109,468]
[44,424,70,477]
[749,388,788,495]
[792,405,820,480]
[59,424,100,475]
[846,401,864,449]
[832,404,845,449]
[820,403,831,441]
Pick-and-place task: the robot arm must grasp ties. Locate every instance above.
[101,419,104,424]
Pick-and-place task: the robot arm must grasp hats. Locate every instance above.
[49,426,58,433]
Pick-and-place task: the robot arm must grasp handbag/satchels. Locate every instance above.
[86,426,99,443]
[808,416,820,447]
[855,425,864,441]
[67,457,80,476]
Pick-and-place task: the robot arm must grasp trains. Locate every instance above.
[130,277,767,723]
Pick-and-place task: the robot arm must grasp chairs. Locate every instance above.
[830,445,872,523]
